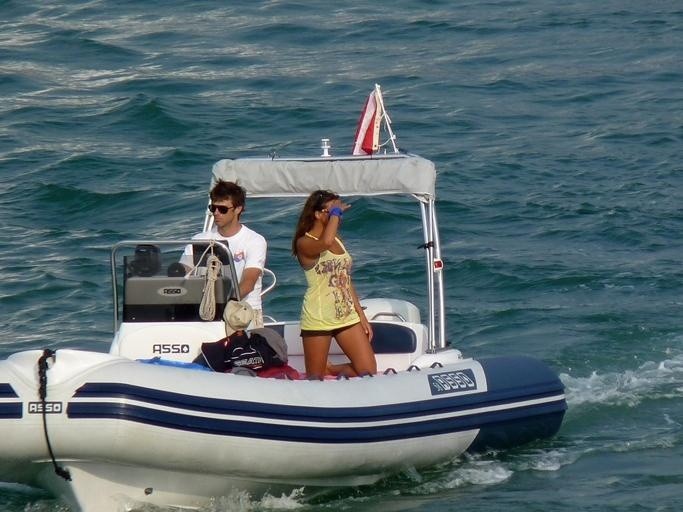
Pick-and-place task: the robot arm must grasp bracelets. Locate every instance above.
[327,206,342,224]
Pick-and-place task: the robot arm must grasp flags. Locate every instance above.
[352,89,381,155]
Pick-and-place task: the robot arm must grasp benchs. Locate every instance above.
[262,311,429,373]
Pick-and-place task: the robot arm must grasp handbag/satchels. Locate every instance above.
[191,330,285,372]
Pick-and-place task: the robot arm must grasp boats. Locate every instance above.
[0,142,566,511]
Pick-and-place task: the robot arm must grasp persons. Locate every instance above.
[291,190,377,381]
[177,179,268,338]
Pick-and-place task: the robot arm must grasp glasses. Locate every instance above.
[208,204,233,213]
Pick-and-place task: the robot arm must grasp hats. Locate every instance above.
[222,297,254,338]
